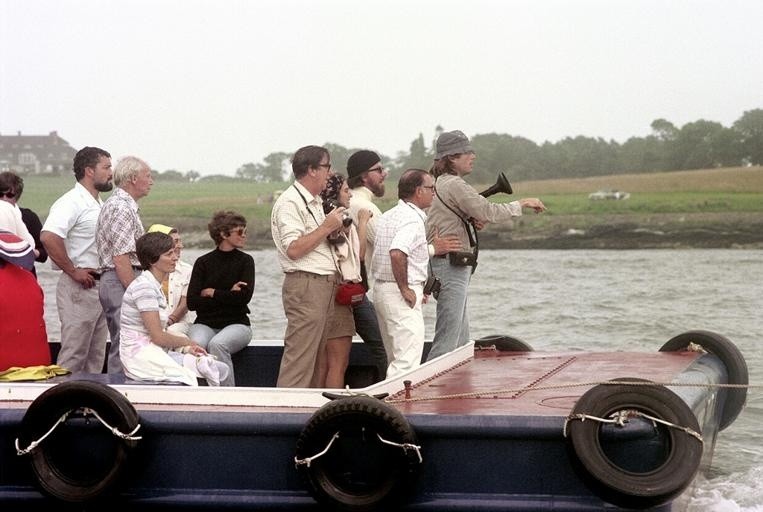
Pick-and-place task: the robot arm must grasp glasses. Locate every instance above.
[370,167,382,174]
[230,229,246,236]
[0,191,15,199]
[424,185,435,190]
[320,164,331,172]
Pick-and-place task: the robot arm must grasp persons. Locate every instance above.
[367,166,438,380]
[117,230,233,387]
[36,144,114,374]
[0,197,53,374]
[93,154,158,380]
[313,174,376,390]
[424,129,546,366]
[184,209,256,387]
[146,223,199,337]
[344,147,465,384]
[268,143,349,389]
[0,170,48,280]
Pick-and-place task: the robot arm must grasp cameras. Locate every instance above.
[322,199,353,228]
[424,277,441,296]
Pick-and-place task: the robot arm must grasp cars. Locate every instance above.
[586,188,628,201]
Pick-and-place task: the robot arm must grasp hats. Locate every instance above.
[433,130,472,161]
[0,203,35,271]
[347,151,380,178]
[148,222,173,236]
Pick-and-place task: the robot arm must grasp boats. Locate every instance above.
[0,331,747,511]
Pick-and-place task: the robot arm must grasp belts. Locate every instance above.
[91,273,101,280]
[133,264,141,271]
